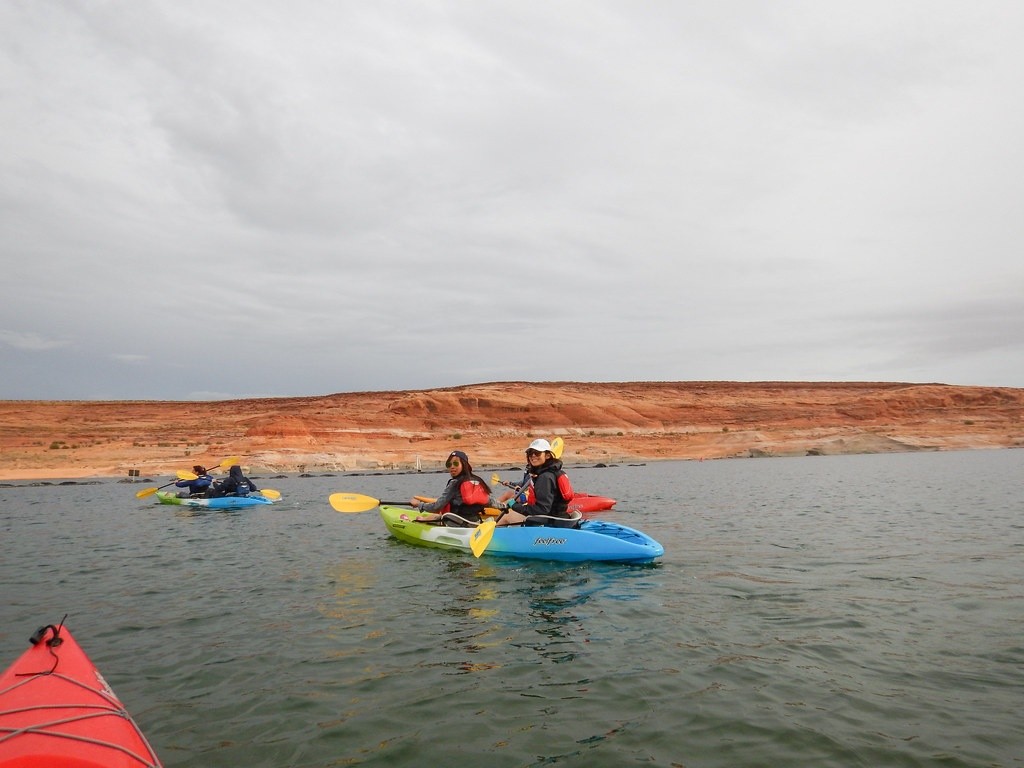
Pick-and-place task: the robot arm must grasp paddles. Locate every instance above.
[177,469,281,498]
[329,492,413,513]
[469,437,564,557]
[137,459,237,500]
[491,473,522,490]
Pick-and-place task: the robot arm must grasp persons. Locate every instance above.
[497,439,569,527]
[410,451,483,522]
[204,466,256,498]
[174,466,213,498]
[496,463,532,503]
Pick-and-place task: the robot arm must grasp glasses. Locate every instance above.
[527,451,542,457]
[446,462,463,468]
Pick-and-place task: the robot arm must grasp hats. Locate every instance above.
[449,451,472,472]
[525,439,551,451]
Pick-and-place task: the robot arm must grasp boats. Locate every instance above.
[376,501,665,564]
[152,489,275,509]
[410,494,619,523]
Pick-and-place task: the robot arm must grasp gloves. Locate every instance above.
[508,498,515,507]
